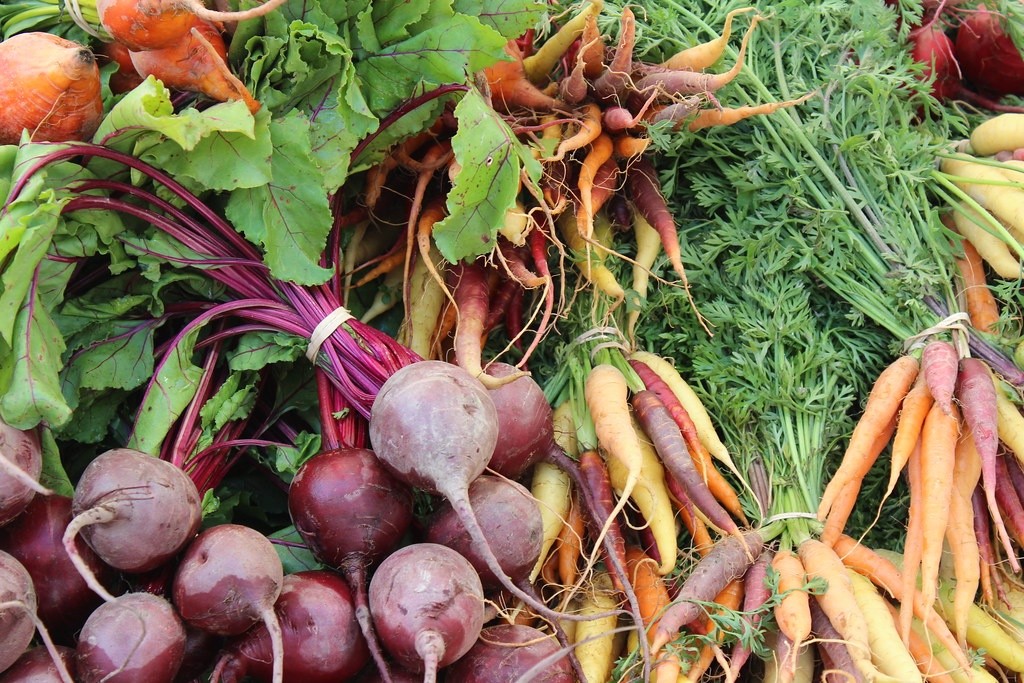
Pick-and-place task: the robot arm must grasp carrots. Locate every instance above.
[0,0,1024,683]
